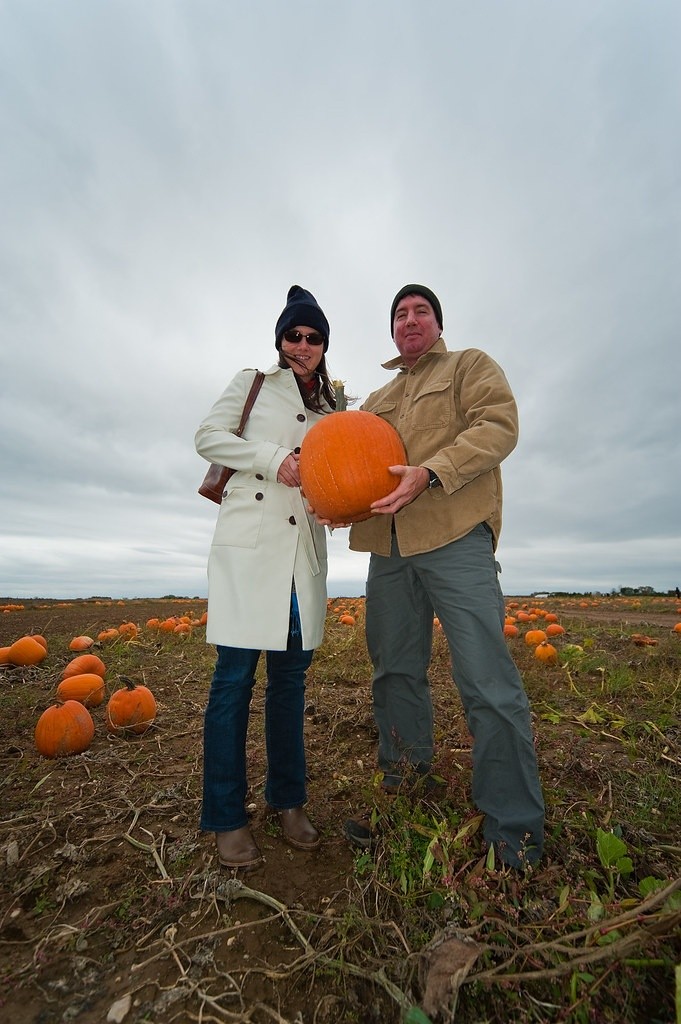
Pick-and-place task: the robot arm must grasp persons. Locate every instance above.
[194,284,336,868]
[299,285,544,883]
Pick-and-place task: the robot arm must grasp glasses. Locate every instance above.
[284,330,325,345]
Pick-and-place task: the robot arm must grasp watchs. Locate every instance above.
[428,468,441,490]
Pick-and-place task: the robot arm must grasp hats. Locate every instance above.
[275,285,330,353]
[391,284,442,338]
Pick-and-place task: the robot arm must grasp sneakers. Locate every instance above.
[347,784,424,846]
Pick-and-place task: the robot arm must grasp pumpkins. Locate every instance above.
[299,381,407,523]
[0,596,681,756]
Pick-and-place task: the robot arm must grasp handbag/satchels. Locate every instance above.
[198,369,266,504]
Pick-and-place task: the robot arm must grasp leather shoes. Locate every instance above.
[264,802,321,850]
[216,827,263,868]
